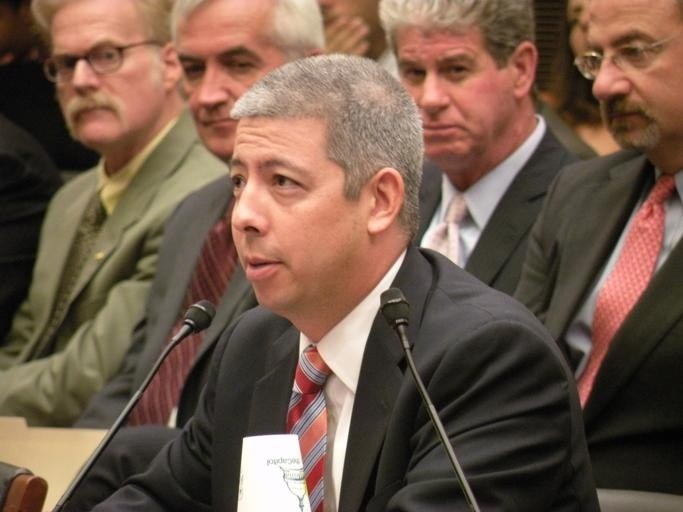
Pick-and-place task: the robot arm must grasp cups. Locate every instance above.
[235,432,311,512]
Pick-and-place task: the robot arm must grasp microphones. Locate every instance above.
[51,299,218,511]
[381,288,479,511]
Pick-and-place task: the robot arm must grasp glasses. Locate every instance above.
[571,37,674,80]
[43,38,166,85]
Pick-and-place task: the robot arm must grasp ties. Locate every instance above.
[125,198,240,426]
[430,196,468,267]
[35,194,108,357]
[285,348,333,511]
[576,173,675,413]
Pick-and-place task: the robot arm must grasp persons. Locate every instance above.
[73,0,329,423]
[317,0,387,62]
[377,1,599,300]
[0,0,231,430]
[92,53,600,512]
[513,0,683,498]
